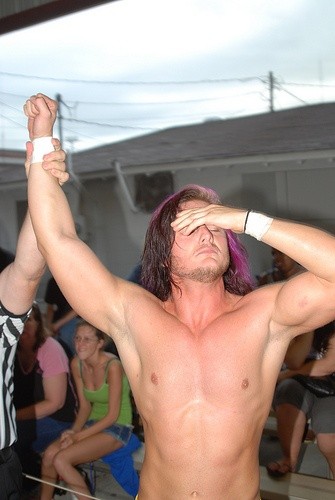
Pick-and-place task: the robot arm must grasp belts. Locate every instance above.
[0,447,12,464]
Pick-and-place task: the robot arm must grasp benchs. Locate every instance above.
[78,440,335,500]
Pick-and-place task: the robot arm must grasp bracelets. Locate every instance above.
[244,210,272,241]
[31,136,54,164]
[242,208,252,231]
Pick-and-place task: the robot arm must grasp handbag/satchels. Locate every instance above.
[292,373,335,397]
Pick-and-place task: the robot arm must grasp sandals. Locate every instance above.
[266,461,289,478]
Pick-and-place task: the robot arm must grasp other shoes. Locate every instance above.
[303,430,315,444]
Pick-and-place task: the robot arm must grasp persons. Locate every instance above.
[24,92,335,500]
[255,245,335,480]
[0,137,69,500]
[13,275,144,500]
